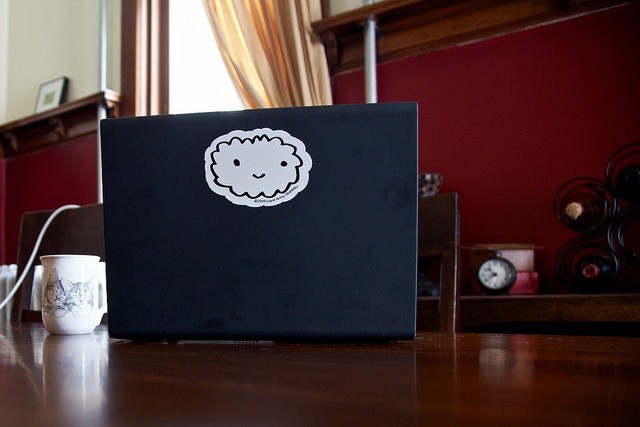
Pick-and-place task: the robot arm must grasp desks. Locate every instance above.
[416,294,640,321]
[2,324,639,426]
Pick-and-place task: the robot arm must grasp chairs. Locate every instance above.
[418,193,460,332]
[11,204,109,324]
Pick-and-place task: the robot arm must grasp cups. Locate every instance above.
[38,254,108,335]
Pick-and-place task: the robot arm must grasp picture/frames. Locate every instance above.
[34,77,69,114]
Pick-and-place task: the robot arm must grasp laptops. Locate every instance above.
[100,101,419,343]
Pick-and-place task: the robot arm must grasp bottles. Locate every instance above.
[572,251,613,287]
[563,194,599,227]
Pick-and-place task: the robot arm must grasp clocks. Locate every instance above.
[478,258,516,292]
